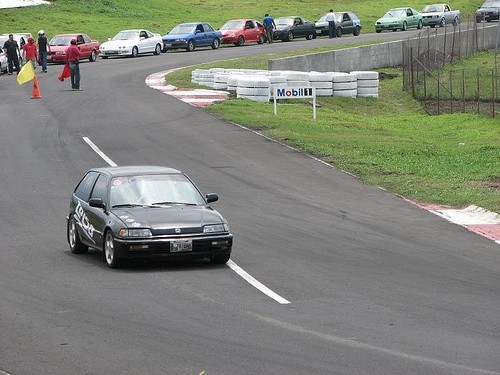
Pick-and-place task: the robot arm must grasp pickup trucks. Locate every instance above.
[420,4,460,27]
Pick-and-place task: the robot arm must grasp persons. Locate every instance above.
[261,13,277,45]
[36,30,52,73]
[63,38,83,92]
[3,34,21,74]
[21,37,38,72]
[325,9,337,39]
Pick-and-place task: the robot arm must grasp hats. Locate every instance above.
[71,39,76,43]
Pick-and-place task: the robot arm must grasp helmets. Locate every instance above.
[27,36,34,41]
[38,30,45,36]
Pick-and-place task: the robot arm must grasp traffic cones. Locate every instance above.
[30,75,42,99]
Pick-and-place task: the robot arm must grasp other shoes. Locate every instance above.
[44,69,47,73]
[74,85,82,91]
[41,70,44,73]
[71,86,74,91]
[8,72,14,75]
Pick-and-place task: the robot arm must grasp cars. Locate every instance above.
[2,34,32,66]
[268,16,317,44]
[98,30,164,59]
[65,163,232,268]
[475,0,500,22]
[0,37,22,74]
[163,21,221,53]
[220,19,266,46]
[374,7,423,31]
[315,10,363,36]
[36,32,100,65]
